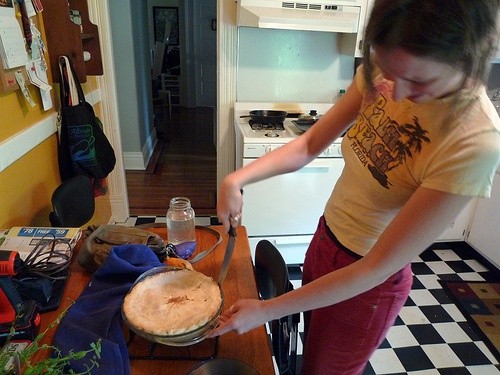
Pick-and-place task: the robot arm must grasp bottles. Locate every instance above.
[166,197,197,259]
[338,89,346,97]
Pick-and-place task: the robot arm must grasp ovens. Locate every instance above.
[236,156,346,265]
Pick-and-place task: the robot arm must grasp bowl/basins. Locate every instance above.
[121,267,226,346]
[187,357,260,375]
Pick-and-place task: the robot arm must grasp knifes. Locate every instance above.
[216,188,243,289]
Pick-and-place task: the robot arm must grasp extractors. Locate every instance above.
[237,0,361,34]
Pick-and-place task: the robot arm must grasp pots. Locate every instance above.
[240,109,288,124]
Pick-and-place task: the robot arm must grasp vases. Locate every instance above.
[153,77,159,98]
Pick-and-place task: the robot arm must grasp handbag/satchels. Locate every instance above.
[55,55,116,198]
[77,223,223,273]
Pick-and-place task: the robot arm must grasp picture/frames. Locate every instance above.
[152,6,179,47]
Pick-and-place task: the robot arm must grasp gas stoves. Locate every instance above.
[233,101,344,158]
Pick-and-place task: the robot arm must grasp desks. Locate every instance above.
[0,211,278,375]
[153,90,172,121]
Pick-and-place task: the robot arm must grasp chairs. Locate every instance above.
[254,240,300,375]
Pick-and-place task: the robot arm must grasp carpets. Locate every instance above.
[437,277,500,363]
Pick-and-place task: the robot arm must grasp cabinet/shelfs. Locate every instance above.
[39,0,104,84]
[162,72,180,105]
[467,169,500,269]
[434,198,476,241]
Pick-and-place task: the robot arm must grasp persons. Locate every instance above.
[205,0,500,375]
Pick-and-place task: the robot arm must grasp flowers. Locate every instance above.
[149,65,161,79]
